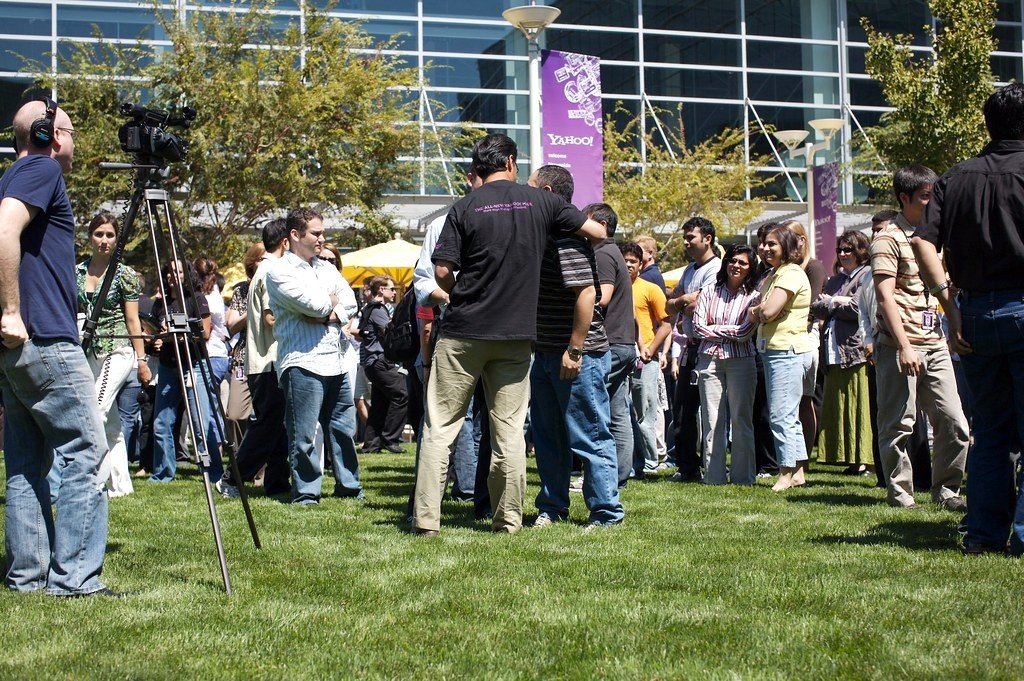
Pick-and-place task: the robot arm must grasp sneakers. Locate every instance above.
[274,487,294,502]
[569,478,583,492]
[216,478,240,499]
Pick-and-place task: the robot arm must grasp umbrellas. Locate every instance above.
[340,233,424,303]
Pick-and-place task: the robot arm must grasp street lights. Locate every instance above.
[771,118,847,261]
[500,5,562,179]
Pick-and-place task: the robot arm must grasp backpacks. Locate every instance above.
[382,289,420,363]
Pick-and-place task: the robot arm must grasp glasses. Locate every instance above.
[835,247,854,254]
[58,126,79,140]
[382,287,396,292]
[318,257,336,264]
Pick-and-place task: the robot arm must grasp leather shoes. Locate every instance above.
[383,444,407,453]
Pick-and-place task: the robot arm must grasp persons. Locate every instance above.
[354,135,673,531]
[75,208,368,502]
[663,218,878,494]
[0,99,123,597]
[870,79,1024,557]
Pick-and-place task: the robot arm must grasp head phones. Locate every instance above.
[11,95,58,155]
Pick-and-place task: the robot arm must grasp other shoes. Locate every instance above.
[666,471,704,483]
[533,515,553,528]
[772,482,807,491]
[76,587,119,599]
[135,468,146,477]
[965,543,988,553]
[843,465,870,477]
[583,519,625,534]
[940,495,967,513]
[617,480,628,489]
[412,526,438,536]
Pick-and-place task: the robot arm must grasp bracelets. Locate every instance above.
[137,354,148,361]
[931,281,949,297]
[751,308,759,318]
[323,315,330,326]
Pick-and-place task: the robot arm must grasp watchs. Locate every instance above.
[567,345,583,357]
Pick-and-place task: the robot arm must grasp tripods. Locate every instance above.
[81,152,263,597]
